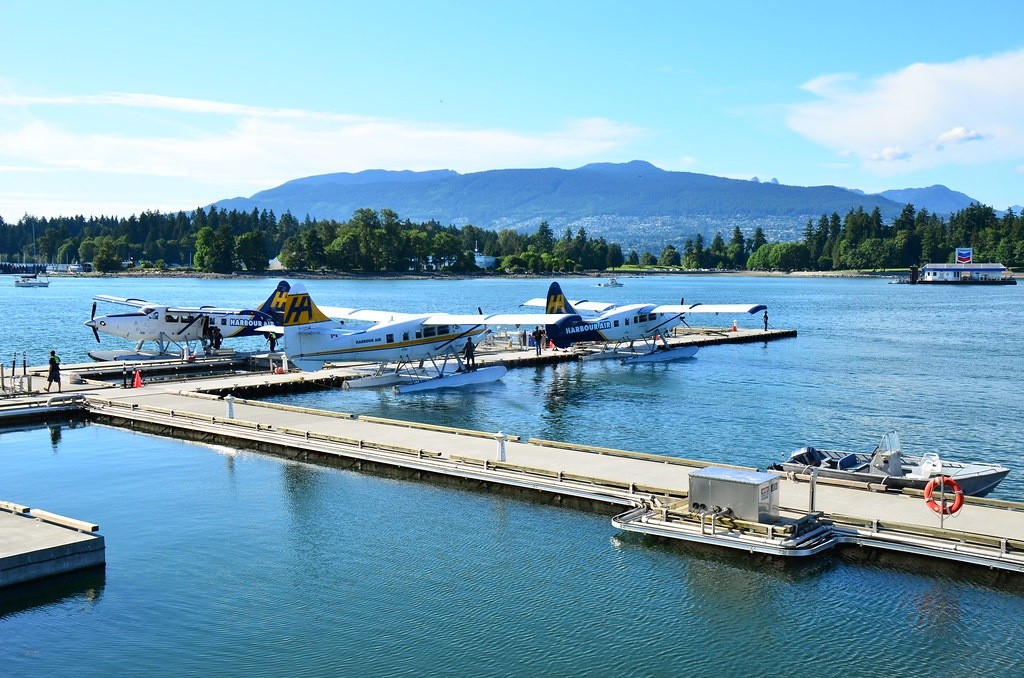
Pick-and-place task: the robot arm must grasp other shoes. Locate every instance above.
[536,354,541,356]
[544,349,547,351]
[44,388,49,391]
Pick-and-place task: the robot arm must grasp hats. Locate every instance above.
[535,326,539,329]
[50,350,55,354]
[541,325,543,327]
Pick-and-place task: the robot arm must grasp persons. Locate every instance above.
[44,350,62,393]
[763,311,768,331]
[207,326,223,349]
[266,333,278,353]
[463,337,477,373]
[48,426,62,453]
[533,325,547,356]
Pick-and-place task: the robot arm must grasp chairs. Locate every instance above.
[904,452,942,479]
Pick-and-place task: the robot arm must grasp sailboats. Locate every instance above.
[15,223,49,287]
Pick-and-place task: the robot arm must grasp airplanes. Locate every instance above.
[83,280,291,361]
[253,281,582,395]
[518,282,768,365]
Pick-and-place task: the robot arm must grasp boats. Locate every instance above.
[780,430,1011,498]
[602,278,624,288]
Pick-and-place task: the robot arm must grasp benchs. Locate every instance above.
[838,453,869,472]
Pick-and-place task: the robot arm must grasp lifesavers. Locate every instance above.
[923,476,964,516]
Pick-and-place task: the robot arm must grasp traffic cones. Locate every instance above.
[133,371,143,387]
